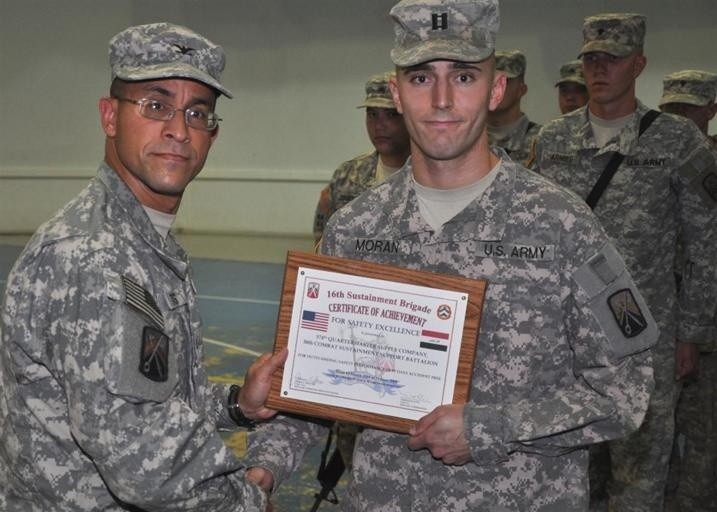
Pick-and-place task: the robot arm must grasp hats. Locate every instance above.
[577,14,645,58]
[555,61,587,87]
[492,49,526,78]
[658,71,717,107]
[390,0,498,63]
[357,71,397,109]
[110,22,234,101]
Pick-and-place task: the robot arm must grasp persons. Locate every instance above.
[482,49,542,174]
[0,21,295,511]
[553,61,586,117]
[661,69,716,511]
[316,1,659,512]
[532,9,714,512]
[307,72,412,244]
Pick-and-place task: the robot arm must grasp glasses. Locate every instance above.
[113,94,224,131]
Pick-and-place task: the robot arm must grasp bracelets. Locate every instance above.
[227,381,254,428]
